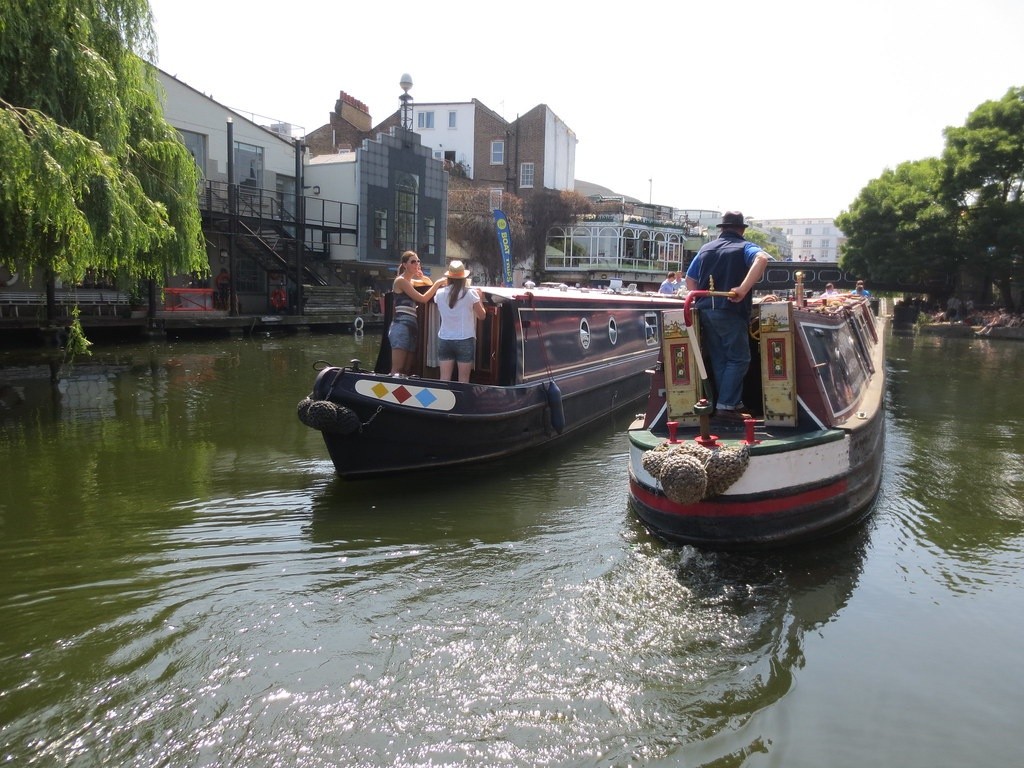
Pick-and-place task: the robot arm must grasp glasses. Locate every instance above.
[406,260,420,264]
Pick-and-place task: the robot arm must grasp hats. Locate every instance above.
[716,210,748,228]
[444,260,470,278]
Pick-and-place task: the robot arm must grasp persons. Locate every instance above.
[658,271,688,294]
[850,279,870,297]
[388,250,447,375]
[930,305,1024,336]
[216,268,230,309]
[685,211,768,420]
[434,260,486,383]
[819,283,838,297]
[785,254,817,262]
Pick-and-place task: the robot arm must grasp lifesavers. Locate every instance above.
[354,317,364,330]
[272,289,286,307]
[355,329,364,337]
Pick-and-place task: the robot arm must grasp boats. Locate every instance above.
[624,269,895,551]
[297,279,686,488]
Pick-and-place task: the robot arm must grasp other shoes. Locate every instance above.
[715,410,752,421]
